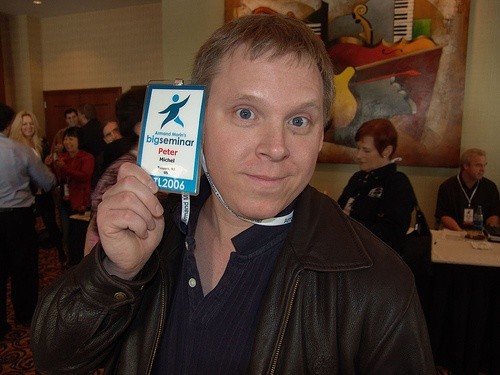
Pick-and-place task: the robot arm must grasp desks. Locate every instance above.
[66,211,91,266]
[406,229,500,268]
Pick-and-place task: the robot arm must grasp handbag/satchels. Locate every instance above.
[390,170,432,289]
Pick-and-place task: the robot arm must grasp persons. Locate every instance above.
[435,149,500,231]
[100,119,122,145]
[45,126,94,266]
[0,103,58,340]
[50,109,82,152]
[336,118,417,250]
[91,86,146,233]
[9,110,67,268]
[78,104,105,188]
[32,12,437,375]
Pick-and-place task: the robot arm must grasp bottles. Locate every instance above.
[473,206,483,231]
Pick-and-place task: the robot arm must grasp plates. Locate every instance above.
[483,226,500,241]
